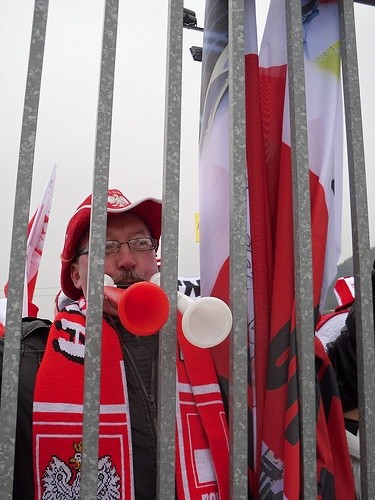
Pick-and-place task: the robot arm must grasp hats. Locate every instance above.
[60,189,166,301]
[333,276,355,312]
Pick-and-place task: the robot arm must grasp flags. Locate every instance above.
[4,164,57,318]
[198,1,356,500]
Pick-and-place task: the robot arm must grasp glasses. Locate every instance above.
[77,237,158,258]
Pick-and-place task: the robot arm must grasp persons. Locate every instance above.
[0,189,375,500]
